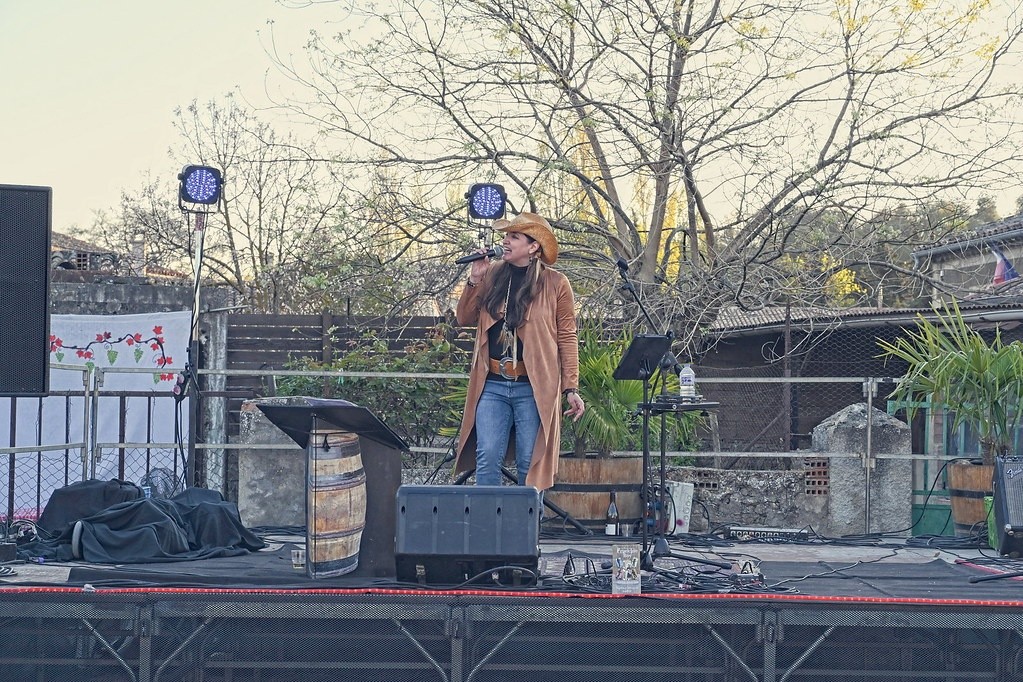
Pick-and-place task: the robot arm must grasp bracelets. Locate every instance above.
[468,278,485,287]
[565,389,579,398]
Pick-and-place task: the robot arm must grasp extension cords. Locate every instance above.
[542,575,576,586]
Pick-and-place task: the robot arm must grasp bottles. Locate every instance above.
[680,364,696,405]
[606,489,619,537]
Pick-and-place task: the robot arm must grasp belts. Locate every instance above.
[489,357,528,380]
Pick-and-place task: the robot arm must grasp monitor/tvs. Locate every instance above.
[612,333,673,380]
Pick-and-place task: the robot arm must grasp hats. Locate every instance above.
[492,212,558,265]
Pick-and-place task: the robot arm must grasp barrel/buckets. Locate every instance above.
[310,430,367,580]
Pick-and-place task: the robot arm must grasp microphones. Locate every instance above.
[454,246,504,264]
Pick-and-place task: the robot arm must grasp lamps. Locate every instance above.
[177,164,224,214]
[463,182,508,227]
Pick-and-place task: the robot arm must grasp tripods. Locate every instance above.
[575,257,733,590]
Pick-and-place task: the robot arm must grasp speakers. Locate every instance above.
[0,184,52,397]
[991,455,1023,559]
[395,485,542,586]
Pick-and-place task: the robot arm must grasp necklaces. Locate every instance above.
[503,273,514,355]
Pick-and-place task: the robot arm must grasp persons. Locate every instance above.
[452,213,585,521]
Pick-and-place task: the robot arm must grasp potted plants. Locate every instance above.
[874,292,1023,536]
[541,325,680,531]
